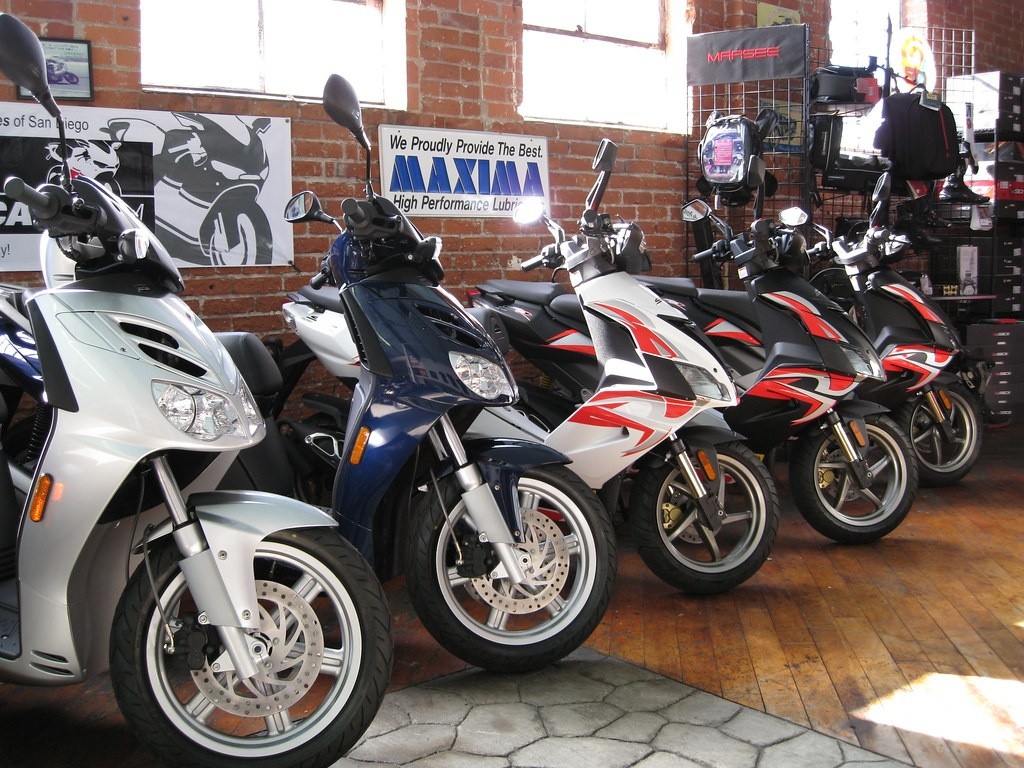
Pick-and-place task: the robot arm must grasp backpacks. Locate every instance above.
[884,83,959,179]
[695,107,779,220]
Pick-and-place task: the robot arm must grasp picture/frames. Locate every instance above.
[15,37,94,102]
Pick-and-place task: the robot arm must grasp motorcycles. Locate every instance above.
[459,138,782,599]
[777,171,987,488]
[635,155,921,546]
[209,73,619,676]
[1,13,395,768]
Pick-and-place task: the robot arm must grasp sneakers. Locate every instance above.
[897,196,951,227]
[940,181,990,203]
